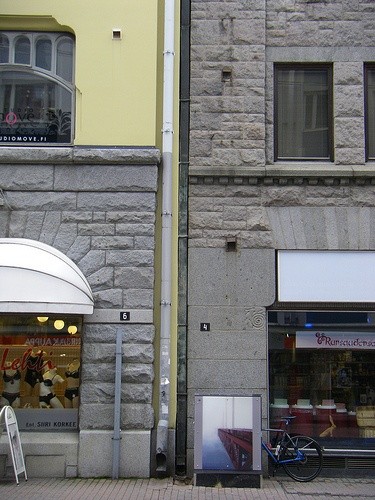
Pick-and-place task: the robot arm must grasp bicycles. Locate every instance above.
[260,415,326,483]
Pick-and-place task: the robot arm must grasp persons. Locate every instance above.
[1,361,23,409]
[64,359,83,408]
[38,361,64,409]
[21,350,46,396]
